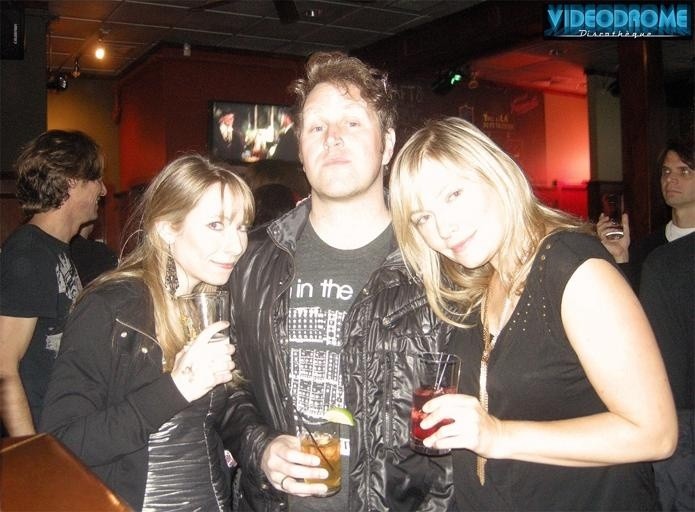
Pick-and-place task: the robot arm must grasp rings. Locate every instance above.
[281,475,295,492]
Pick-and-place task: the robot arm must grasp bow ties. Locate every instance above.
[277,132,284,140]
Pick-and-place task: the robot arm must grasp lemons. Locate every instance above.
[320,409,355,426]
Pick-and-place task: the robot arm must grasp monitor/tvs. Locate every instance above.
[207,97,309,168]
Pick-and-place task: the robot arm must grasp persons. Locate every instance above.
[213,110,241,161]
[216,48,466,512]
[388,116,679,512]
[38,152,256,511]
[595,138,695,512]
[246,182,304,230]
[70,220,103,286]
[271,109,299,161]
[0,129,108,437]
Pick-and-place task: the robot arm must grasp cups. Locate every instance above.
[177,292,230,342]
[602,192,625,240]
[407,353,460,459]
[297,405,341,498]
